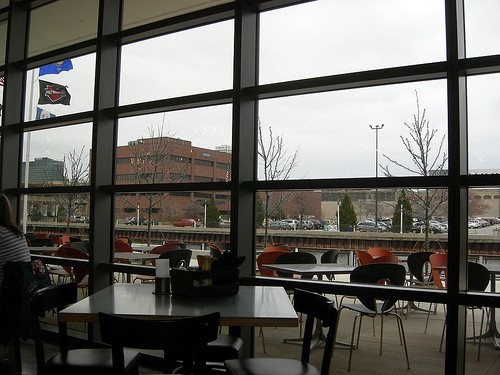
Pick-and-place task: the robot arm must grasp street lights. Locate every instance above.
[369,123,385,227]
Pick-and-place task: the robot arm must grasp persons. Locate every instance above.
[0,192,31,375]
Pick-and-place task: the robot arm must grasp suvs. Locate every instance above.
[309,220,318,230]
[357,220,387,233]
[468,219,480,229]
[281,219,300,230]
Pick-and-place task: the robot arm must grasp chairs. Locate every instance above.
[0,233,500,375]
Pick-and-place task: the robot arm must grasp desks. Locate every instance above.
[58,282,298,374]
[433,264,500,349]
[262,263,357,353]
[115,252,161,283]
[29,247,59,256]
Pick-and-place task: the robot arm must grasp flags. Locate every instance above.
[39,59,73,76]
[36,107,56,120]
[0,76,4,86]
[38,79,71,105]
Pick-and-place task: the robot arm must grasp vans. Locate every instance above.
[316,220,322,229]
[298,220,313,230]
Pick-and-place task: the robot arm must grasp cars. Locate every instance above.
[410,224,439,234]
[261,222,266,228]
[172,218,196,228]
[269,221,292,230]
[124,216,142,226]
